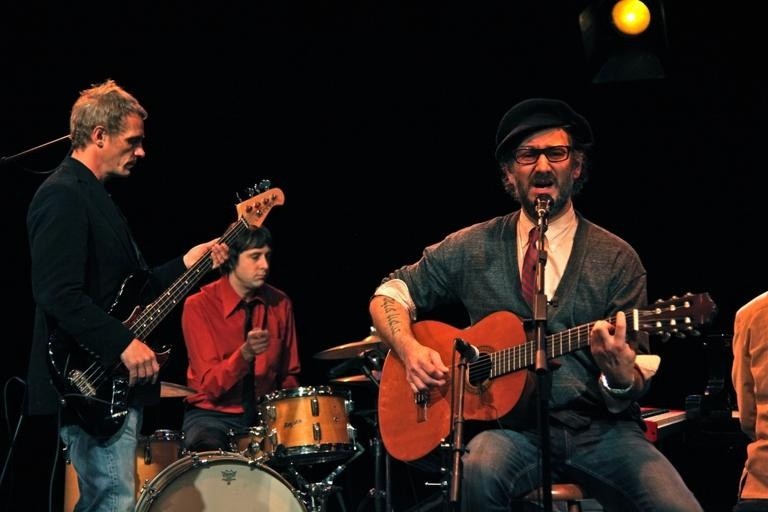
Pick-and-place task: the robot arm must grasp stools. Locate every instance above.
[527,484,584,510]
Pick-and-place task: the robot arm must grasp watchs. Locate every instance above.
[602,372,638,397]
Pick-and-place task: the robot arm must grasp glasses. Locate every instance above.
[508,142,576,164]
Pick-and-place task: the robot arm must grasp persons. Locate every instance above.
[182,221,320,481]
[732,291,768,511]
[370,101,705,510]
[27,82,230,512]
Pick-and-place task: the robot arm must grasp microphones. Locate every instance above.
[534,193,554,233]
[454,338,479,363]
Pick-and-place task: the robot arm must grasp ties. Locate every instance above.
[239,299,258,409]
[520,227,546,312]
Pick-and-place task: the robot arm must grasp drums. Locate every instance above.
[64,435,179,512]
[132,450,314,511]
[248,384,361,463]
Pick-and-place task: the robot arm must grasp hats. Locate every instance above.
[489,95,576,161]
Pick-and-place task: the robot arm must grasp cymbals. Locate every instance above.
[160,381,197,398]
[332,371,380,383]
[319,335,383,360]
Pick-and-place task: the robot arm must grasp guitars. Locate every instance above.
[377,291,716,463]
[46,178,285,448]
